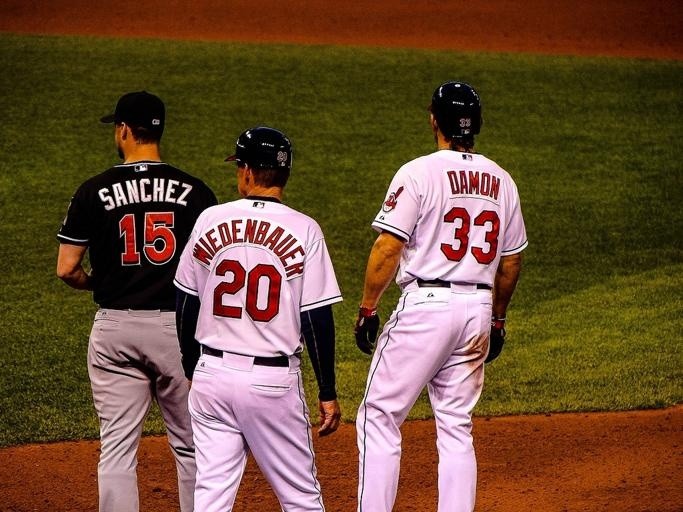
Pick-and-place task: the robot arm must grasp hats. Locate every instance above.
[100,91,165,129]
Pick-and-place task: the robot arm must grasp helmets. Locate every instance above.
[426,82,483,137]
[224,127,292,169]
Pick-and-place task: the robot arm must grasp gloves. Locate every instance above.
[485,315,506,363]
[355,303,380,355]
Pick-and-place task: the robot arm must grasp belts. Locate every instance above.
[416,277,492,290]
[201,343,289,367]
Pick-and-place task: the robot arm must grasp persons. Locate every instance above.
[351,81,528,512]
[173,126,343,509]
[57,90,218,511]
